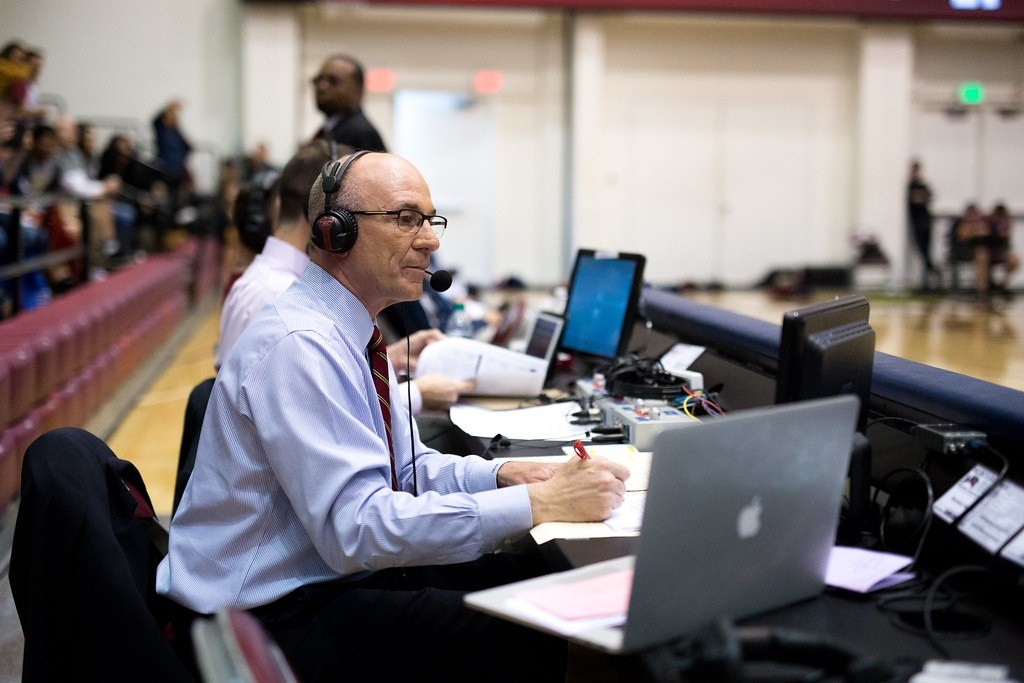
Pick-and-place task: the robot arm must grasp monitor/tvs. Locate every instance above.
[558,249,646,389]
[774,291,876,408]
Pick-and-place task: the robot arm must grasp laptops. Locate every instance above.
[467,393,864,657]
[522,310,566,387]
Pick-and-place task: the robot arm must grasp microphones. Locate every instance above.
[424,270,452,292]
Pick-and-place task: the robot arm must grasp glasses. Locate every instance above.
[349,209,447,238]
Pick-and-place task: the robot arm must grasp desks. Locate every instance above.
[452,386,1024,683]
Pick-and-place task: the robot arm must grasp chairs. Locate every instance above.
[22,375,298,683]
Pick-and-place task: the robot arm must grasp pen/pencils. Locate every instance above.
[573,440,590,460]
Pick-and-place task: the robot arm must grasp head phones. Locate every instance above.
[312,149,372,251]
[604,350,694,401]
[243,172,278,254]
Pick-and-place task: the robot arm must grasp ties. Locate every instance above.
[366,325,399,492]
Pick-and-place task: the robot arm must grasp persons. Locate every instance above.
[152,152,629,683]
[908,162,943,278]
[0,40,287,324]
[950,203,1020,315]
[203,55,531,417]
